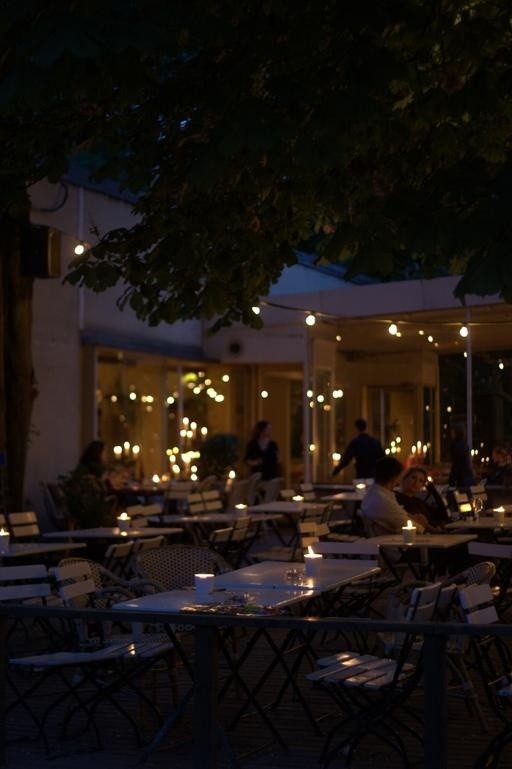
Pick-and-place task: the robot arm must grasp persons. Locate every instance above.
[65,440,144,586]
[479,445,512,486]
[393,465,448,581]
[360,454,470,584]
[243,419,281,538]
[328,418,389,486]
[444,439,478,512]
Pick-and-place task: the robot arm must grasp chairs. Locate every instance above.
[1,478,512,768]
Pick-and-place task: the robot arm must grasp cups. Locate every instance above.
[303,554,323,578]
[0,531,10,550]
[402,526,417,543]
[235,505,247,517]
[493,508,505,522]
[117,516,131,531]
[194,573,215,602]
